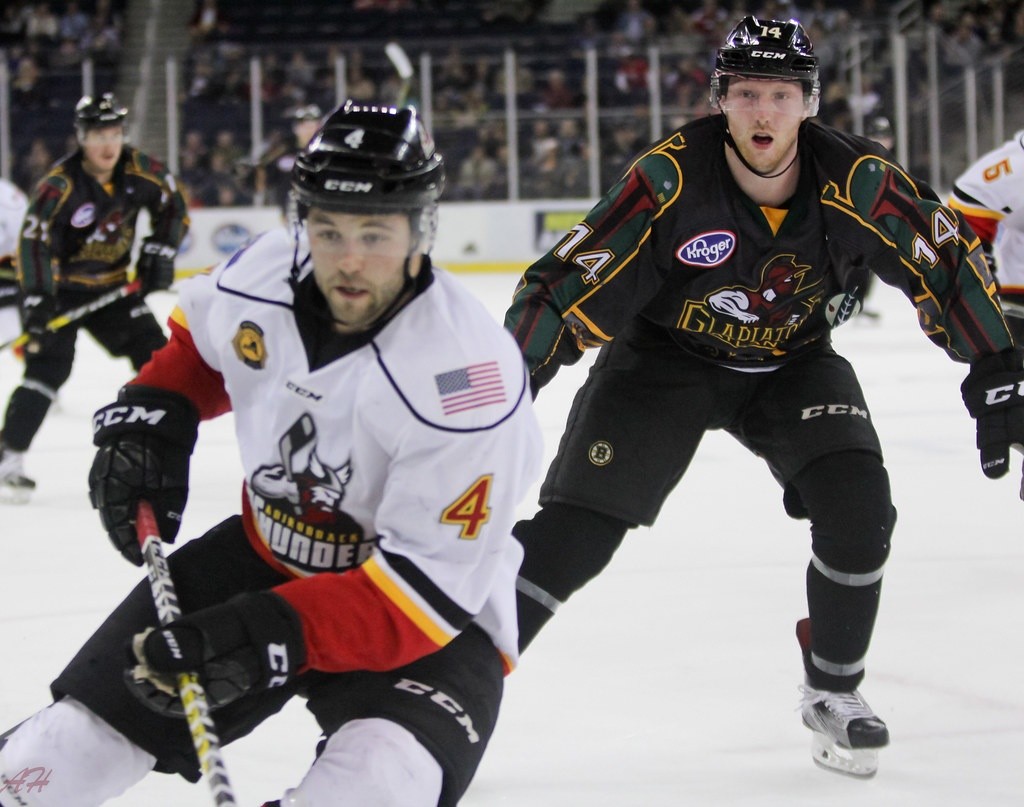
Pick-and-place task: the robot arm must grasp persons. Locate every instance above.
[504,12,1023,776]
[1,91,192,504]
[0,0,1024,358]
[0,96,535,807]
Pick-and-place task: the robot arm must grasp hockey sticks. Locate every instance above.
[0,277,145,354]
[130,496,248,807]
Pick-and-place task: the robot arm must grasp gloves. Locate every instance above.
[137,237,178,290]
[122,591,307,720]
[88,387,201,567]
[960,344,1024,500]
[19,291,59,347]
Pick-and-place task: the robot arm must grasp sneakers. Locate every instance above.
[796,618,889,780]
[0,430,35,504]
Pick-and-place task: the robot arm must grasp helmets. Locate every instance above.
[714,16,818,96]
[291,99,446,213]
[76,92,128,127]
